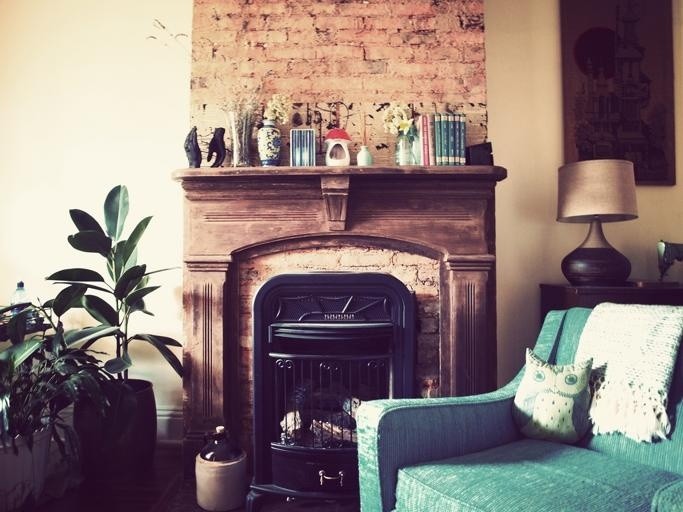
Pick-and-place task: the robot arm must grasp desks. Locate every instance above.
[535,278,683,330]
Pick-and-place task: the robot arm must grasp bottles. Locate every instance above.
[200,426,241,461]
[355,145,370,167]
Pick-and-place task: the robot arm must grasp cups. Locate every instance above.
[195,450,246,510]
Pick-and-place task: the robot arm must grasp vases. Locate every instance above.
[391,135,415,167]
[221,109,255,168]
[253,118,284,167]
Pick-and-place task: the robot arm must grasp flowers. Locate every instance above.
[260,93,290,125]
[381,99,419,146]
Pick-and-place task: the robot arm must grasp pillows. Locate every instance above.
[510,343,601,448]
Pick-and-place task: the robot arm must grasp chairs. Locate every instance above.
[350,300,683,512]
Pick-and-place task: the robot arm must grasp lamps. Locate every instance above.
[548,157,645,287]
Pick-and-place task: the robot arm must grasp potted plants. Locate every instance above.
[0,297,127,512]
[37,179,190,468]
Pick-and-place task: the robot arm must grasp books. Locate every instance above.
[417,113,467,166]
[290,129,316,166]
